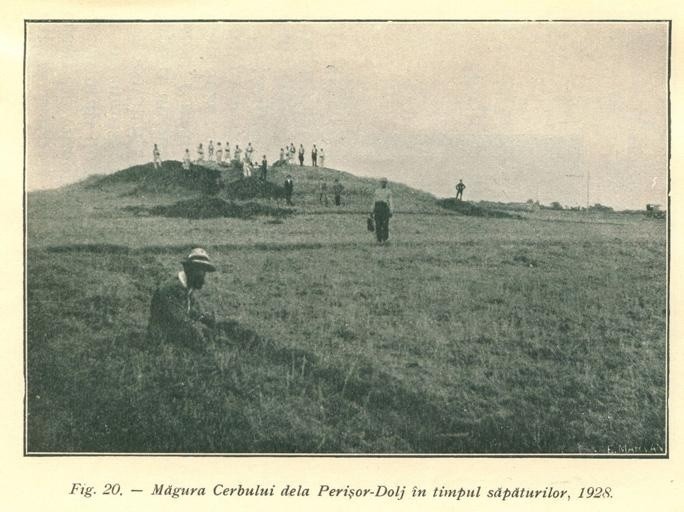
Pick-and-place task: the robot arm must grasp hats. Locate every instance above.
[179,248,216,272]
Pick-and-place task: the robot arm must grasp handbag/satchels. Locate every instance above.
[367,217,374,232]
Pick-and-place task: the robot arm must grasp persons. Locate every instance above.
[147,248,218,352]
